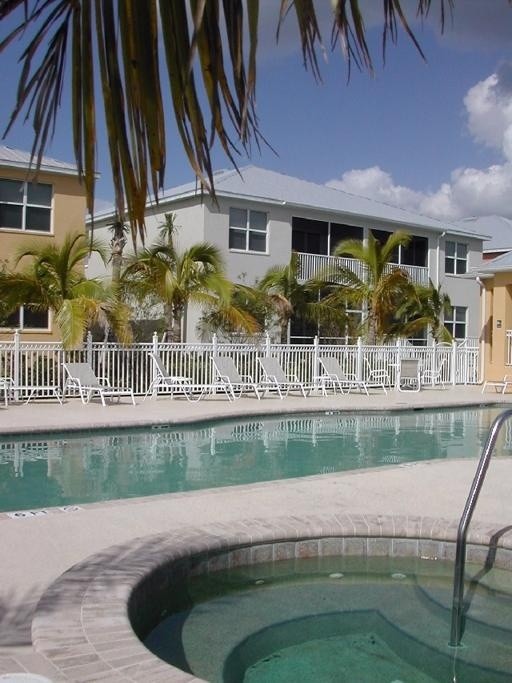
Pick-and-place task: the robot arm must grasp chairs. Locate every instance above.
[255,353,330,399]
[58,359,139,409]
[360,353,446,392]
[311,351,371,400]
[482,375,512,395]
[142,352,236,405]
[0,376,67,410]
[208,354,290,405]
